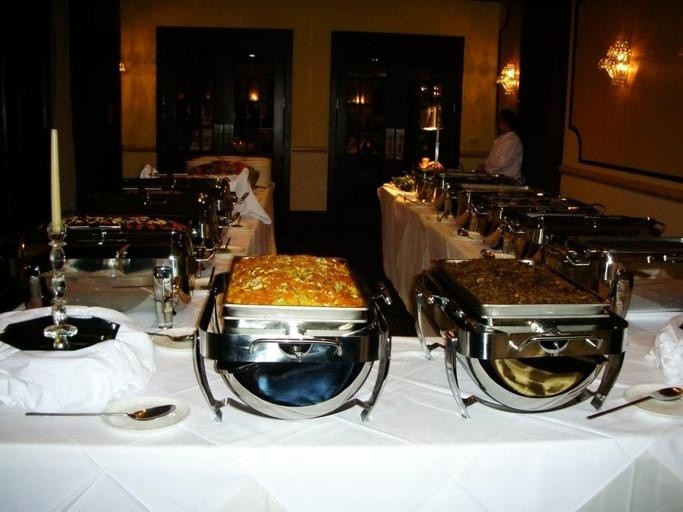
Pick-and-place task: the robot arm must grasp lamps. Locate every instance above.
[421,105,443,169]
[598,36,632,85]
[496,64,521,96]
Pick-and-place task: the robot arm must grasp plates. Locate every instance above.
[102,395,190,428]
[624,382,682,419]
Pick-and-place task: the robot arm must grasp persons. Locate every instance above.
[474,107,523,182]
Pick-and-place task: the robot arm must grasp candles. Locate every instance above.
[51,128,63,232]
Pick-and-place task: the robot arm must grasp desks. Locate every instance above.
[378,183,681,324]
[1,328,683,511]
[187,156,272,187]
[12,184,278,327]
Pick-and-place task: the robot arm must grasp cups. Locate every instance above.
[151,267,176,330]
[19,266,43,310]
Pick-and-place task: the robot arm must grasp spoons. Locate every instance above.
[146,330,192,344]
[587,386,682,421]
[21,403,177,422]
